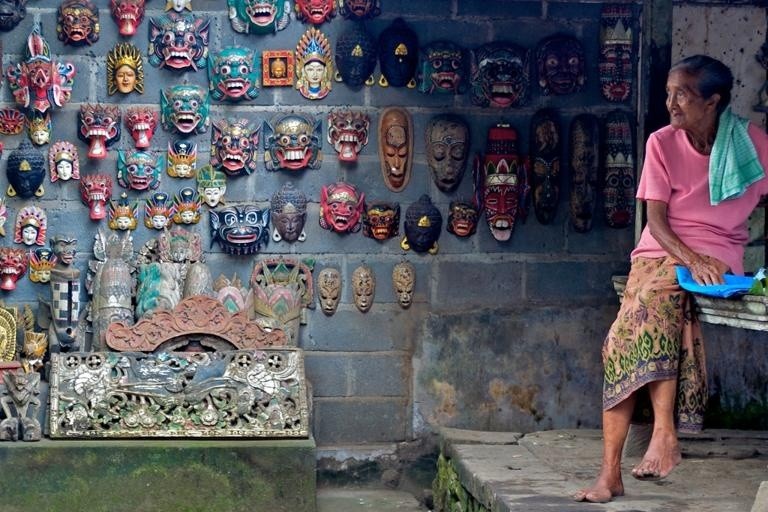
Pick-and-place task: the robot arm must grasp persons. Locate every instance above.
[30,129,50,147]
[113,64,137,94]
[204,187,220,207]
[175,162,191,177]
[271,59,286,78]
[572,54,767,504]
[5,138,46,199]
[334,21,377,89]
[376,18,419,90]
[172,0,186,13]
[21,224,39,246]
[114,216,132,231]
[34,269,52,283]
[170,246,188,264]
[55,159,74,181]
[180,211,195,224]
[149,215,170,229]
[300,59,330,100]
